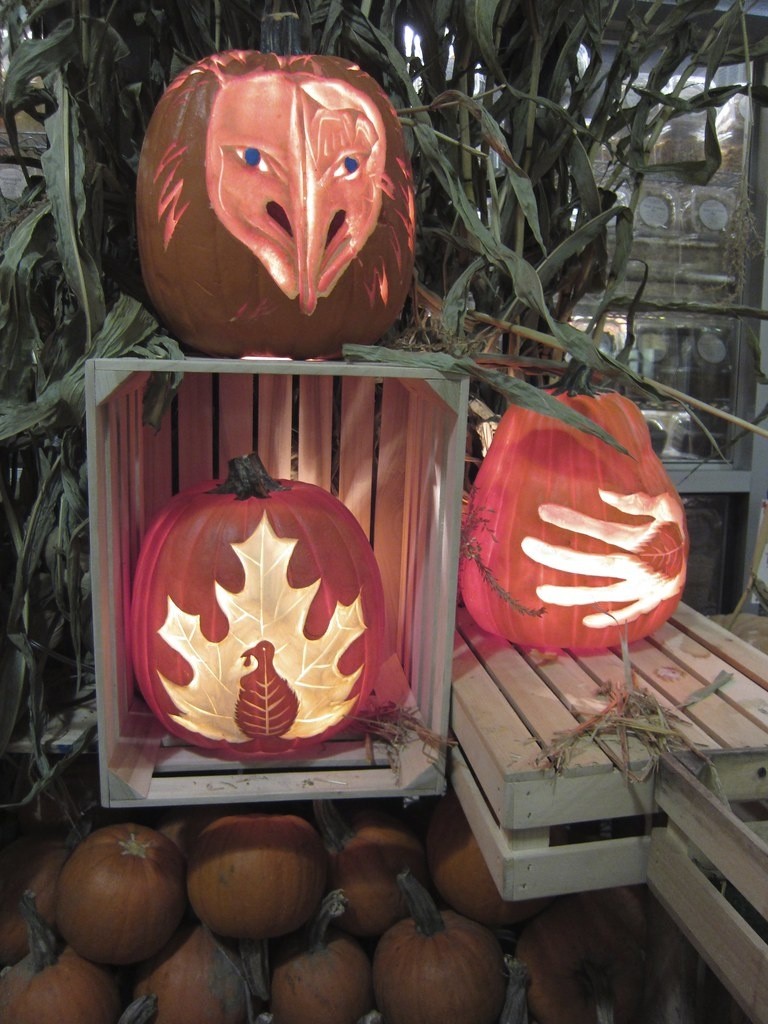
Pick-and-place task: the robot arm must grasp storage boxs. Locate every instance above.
[80,354,768,1022]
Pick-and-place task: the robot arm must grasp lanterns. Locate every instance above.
[127,455,387,763]
[459,357,689,652]
[134,12,416,365]
